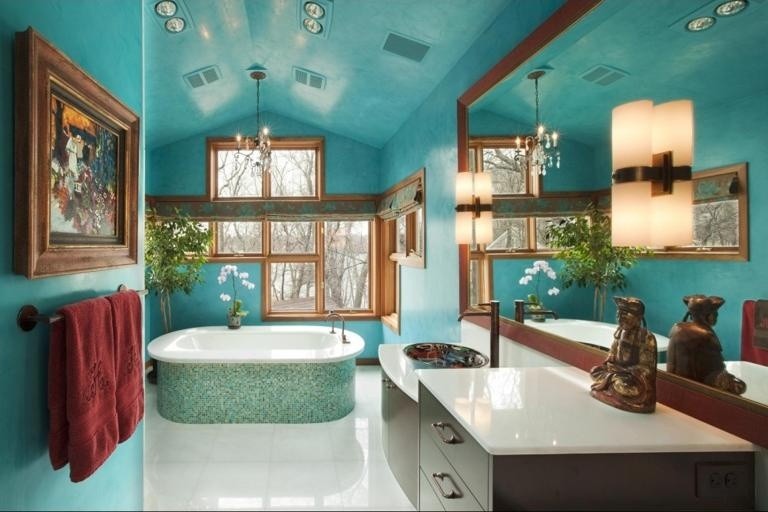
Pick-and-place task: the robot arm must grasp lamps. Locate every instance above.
[607,97,654,252]
[654,100,699,248]
[454,172,473,246]
[236,71,274,163]
[512,69,561,178]
[473,174,493,246]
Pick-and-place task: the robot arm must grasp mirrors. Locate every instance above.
[457,2,766,453]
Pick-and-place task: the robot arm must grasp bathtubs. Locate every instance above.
[536,317,670,353]
[147,324,365,364]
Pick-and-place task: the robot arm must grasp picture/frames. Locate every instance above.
[10,26,141,279]
[604,162,750,262]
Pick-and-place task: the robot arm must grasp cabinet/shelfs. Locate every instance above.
[418,382,757,510]
[378,362,416,510]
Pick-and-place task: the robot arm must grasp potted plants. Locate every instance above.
[142,203,211,385]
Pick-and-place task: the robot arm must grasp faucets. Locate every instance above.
[458,300,500,367]
[515,299,559,323]
[324,313,351,345]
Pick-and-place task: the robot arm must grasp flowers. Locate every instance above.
[516,258,561,307]
[217,263,254,318]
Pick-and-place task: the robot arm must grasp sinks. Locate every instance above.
[397,341,491,377]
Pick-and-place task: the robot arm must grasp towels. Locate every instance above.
[47,299,117,483]
[738,298,764,364]
[105,288,145,446]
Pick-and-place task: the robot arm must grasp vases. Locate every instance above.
[528,302,548,322]
[227,311,241,329]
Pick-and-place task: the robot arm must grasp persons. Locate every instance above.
[589,295,656,413]
[666,294,747,396]
